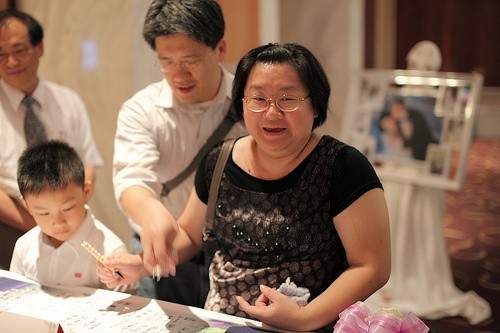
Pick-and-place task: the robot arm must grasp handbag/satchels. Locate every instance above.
[196,137,236,308]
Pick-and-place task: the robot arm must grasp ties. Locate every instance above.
[22,96,48,147]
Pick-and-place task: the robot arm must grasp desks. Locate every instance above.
[1,269,302,333]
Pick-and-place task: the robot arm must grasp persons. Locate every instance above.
[378,98,440,160]
[9,139,139,294]
[112,0,250,310]
[0,9,105,271]
[96,44,391,331]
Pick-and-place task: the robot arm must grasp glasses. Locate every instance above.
[242,94,311,113]
[154,51,214,73]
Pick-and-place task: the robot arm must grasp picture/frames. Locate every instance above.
[341,70,484,190]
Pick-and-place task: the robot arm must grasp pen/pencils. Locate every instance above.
[81,242,124,279]
